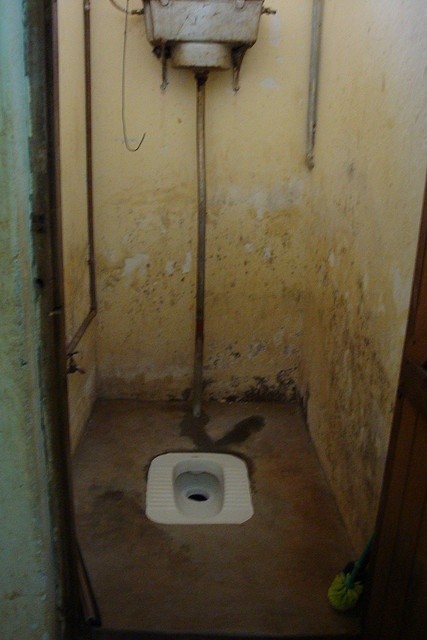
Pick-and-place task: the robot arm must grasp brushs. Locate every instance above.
[327,534,373,612]
